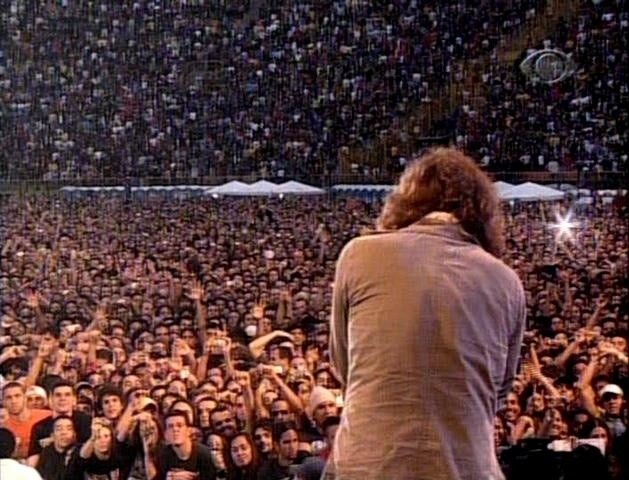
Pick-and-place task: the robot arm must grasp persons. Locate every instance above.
[0,0,628,479]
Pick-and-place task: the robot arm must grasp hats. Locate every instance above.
[309,384,335,418]
[137,397,158,412]
[25,384,47,408]
[597,384,623,396]
[73,382,94,389]
